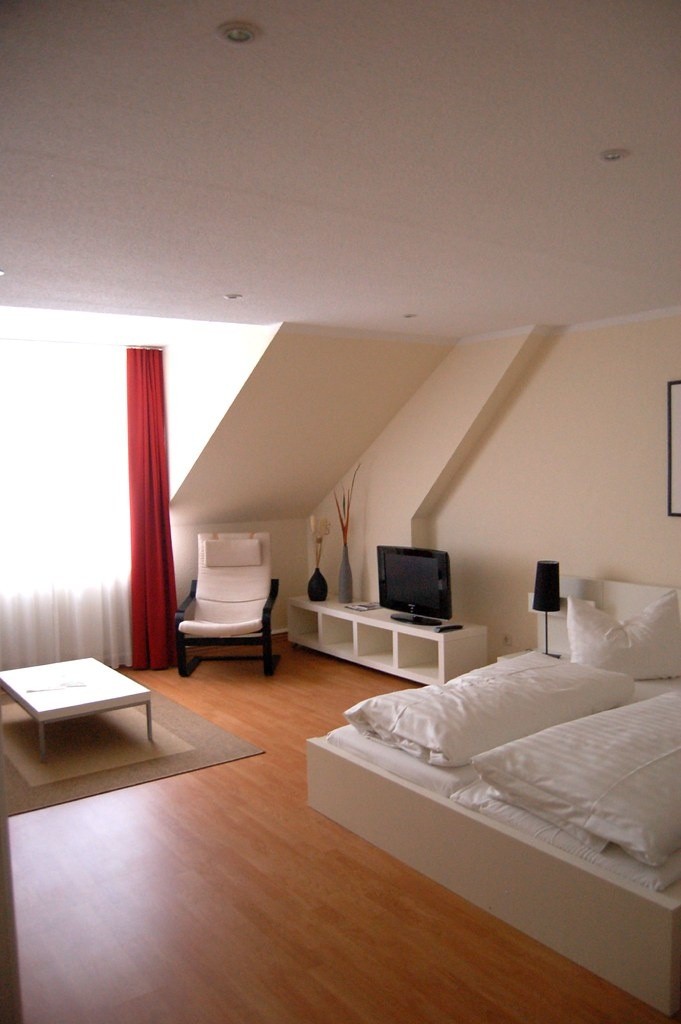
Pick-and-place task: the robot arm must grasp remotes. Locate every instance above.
[435,625,463,633]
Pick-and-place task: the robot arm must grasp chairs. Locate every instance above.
[176,532,282,677]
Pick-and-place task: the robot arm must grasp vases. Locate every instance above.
[337,544,353,603]
[307,567,328,602]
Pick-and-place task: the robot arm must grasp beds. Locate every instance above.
[305,573,681,1018]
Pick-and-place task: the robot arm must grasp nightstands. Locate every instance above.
[496,647,571,663]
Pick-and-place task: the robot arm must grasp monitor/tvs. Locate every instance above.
[377,545,452,626]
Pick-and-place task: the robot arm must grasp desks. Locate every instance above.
[0,657,153,762]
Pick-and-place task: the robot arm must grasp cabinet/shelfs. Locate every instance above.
[286,593,489,684]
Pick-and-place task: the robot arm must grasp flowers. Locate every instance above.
[309,513,332,568]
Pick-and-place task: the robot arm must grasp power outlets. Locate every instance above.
[502,632,513,646]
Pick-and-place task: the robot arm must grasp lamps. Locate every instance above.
[533,560,561,659]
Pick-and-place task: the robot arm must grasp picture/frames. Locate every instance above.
[667,380,681,516]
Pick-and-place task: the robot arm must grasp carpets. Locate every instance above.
[0,671,267,816]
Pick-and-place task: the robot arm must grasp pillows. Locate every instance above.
[566,589,681,679]
